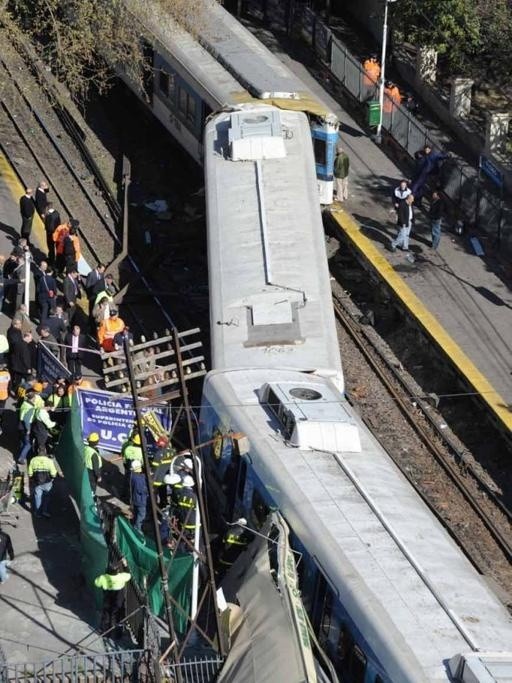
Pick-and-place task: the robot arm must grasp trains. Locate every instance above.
[62,1,511,683]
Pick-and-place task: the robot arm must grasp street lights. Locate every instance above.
[375,0,399,144]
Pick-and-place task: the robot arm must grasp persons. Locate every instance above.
[4,368,246,633]
[384,144,450,253]
[363,56,401,112]
[334,147,349,202]
[2,181,134,391]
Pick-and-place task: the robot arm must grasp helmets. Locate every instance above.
[32,383,42,392]
[239,518,247,525]
[87,432,195,487]
